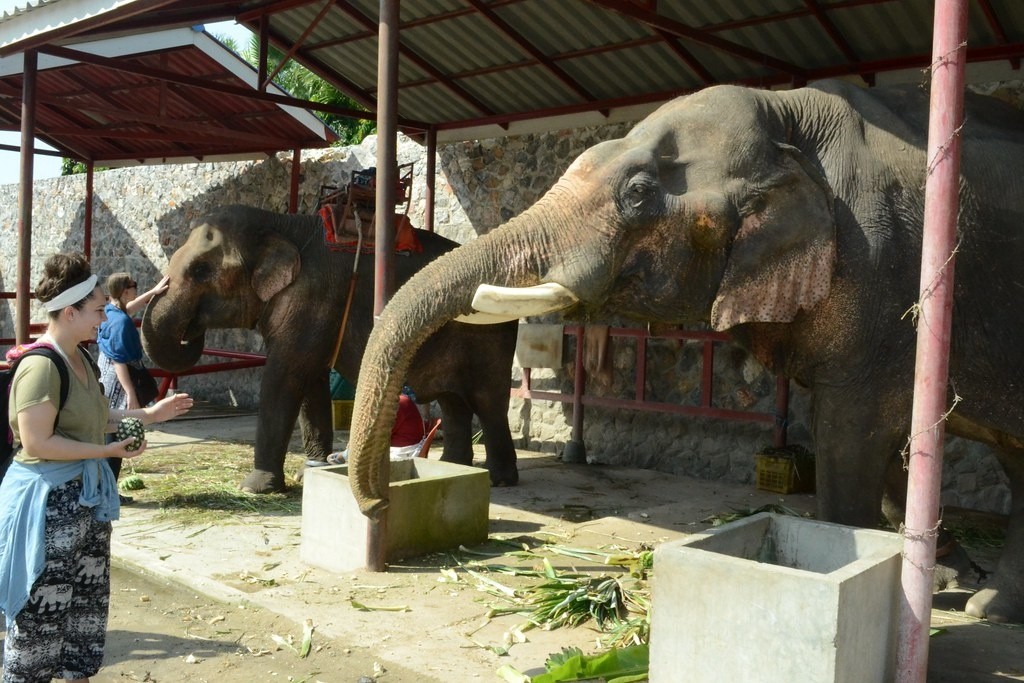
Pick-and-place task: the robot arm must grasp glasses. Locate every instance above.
[126,281,137,289]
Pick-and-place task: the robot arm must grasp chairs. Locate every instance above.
[419,415,442,458]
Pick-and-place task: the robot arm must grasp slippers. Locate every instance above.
[327,452,344,465]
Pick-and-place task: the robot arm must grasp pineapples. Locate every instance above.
[117,417,145,452]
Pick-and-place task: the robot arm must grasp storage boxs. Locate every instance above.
[756,455,814,495]
[330,371,355,430]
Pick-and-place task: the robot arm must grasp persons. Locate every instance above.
[327,394,426,465]
[97,273,170,505]
[0,255,193,683]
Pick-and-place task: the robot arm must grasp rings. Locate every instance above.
[176,407,178,410]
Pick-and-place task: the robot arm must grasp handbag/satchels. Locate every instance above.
[127,363,159,406]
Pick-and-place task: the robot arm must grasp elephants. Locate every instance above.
[345,76,1024,626]
[139,203,521,494]
